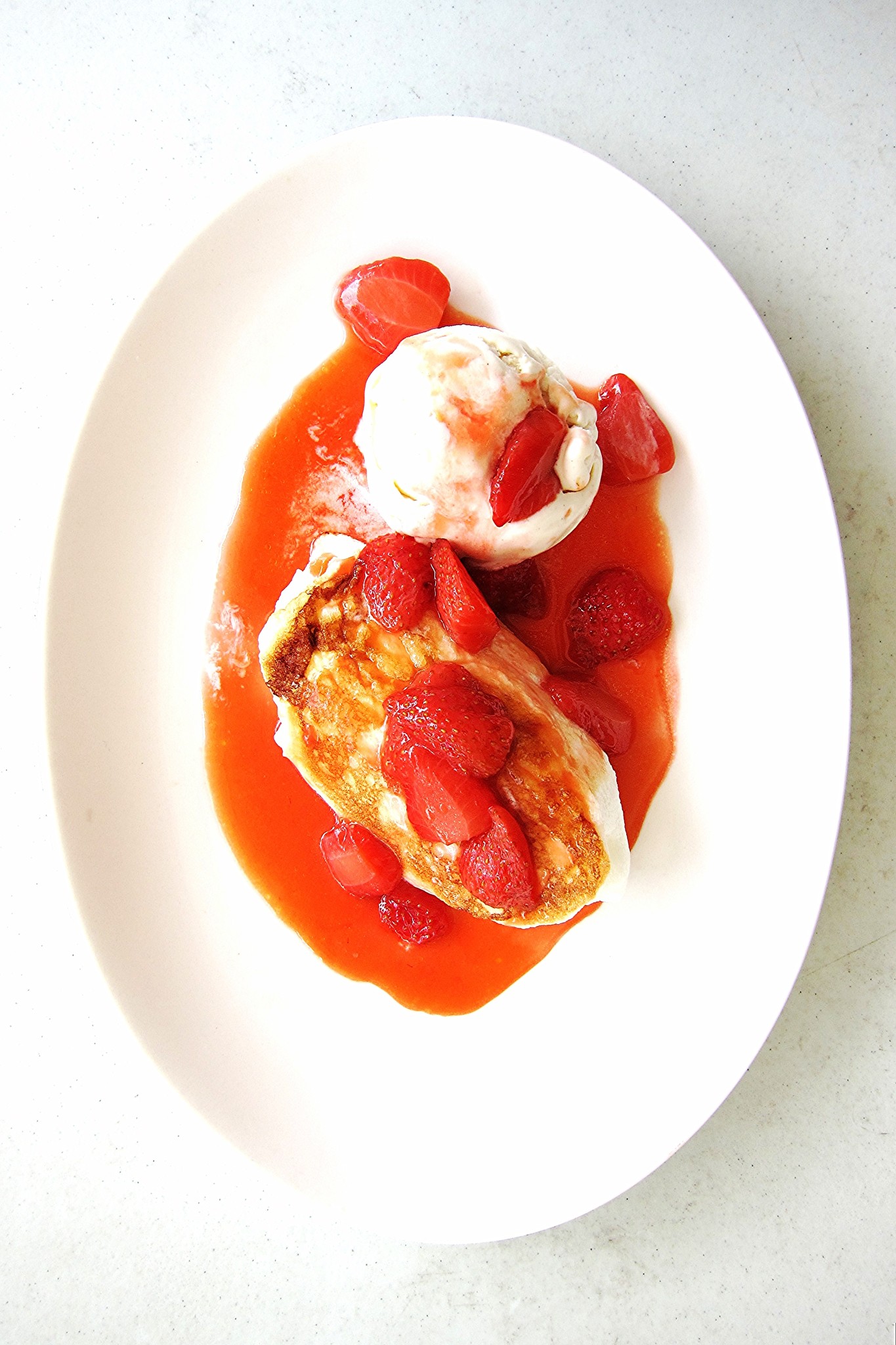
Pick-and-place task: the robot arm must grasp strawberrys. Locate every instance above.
[317,262,675,946]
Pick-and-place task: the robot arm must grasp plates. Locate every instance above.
[45,119,855,1246]
